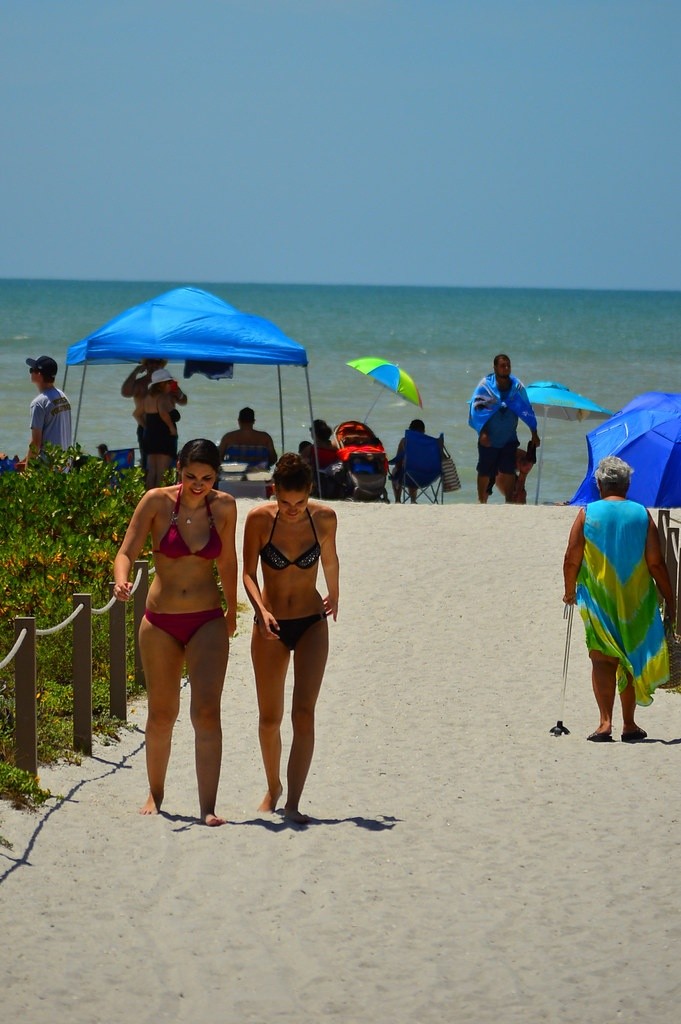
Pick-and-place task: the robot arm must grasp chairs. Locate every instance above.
[104,448,135,487]
[215,444,274,491]
[0,457,18,475]
[387,429,444,504]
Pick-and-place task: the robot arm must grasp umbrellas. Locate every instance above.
[467,379,616,503]
[346,355,423,424]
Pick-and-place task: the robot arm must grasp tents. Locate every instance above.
[570,389,681,506]
[61,287,322,499]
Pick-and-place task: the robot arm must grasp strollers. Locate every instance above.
[332,419,390,504]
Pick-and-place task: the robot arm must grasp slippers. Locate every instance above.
[622,727,647,742]
[587,732,612,742]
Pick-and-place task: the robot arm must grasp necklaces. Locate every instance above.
[176,501,199,524]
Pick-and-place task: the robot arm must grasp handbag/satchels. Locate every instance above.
[438,436,461,492]
[657,617,681,688]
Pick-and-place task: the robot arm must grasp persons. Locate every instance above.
[113,438,239,826]
[25,356,72,474]
[99,355,544,507]
[562,457,675,741]
[241,453,340,823]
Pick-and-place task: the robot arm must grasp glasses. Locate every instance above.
[30,368,43,375]
[146,359,160,365]
[161,382,171,385]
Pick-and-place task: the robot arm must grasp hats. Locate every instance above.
[141,358,166,369]
[26,356,57,377]
[147,369,173,391]
